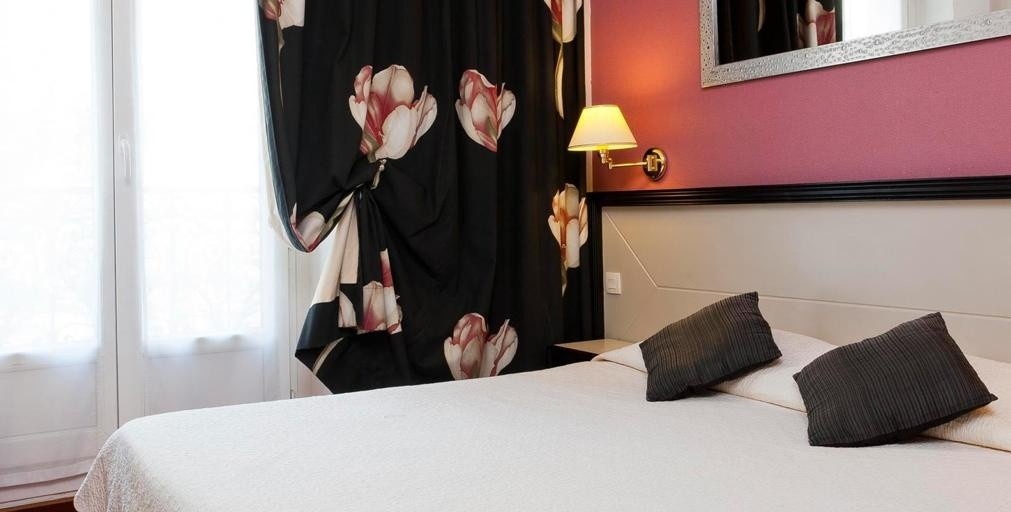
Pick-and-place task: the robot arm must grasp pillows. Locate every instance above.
[791,311,999,446]
[638,290,783,402]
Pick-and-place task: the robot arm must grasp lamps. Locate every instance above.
[568,101,665,179]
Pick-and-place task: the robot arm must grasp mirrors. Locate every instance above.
[694,2,1011,89]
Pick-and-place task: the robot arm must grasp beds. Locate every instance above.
[70,172,1011,509]
